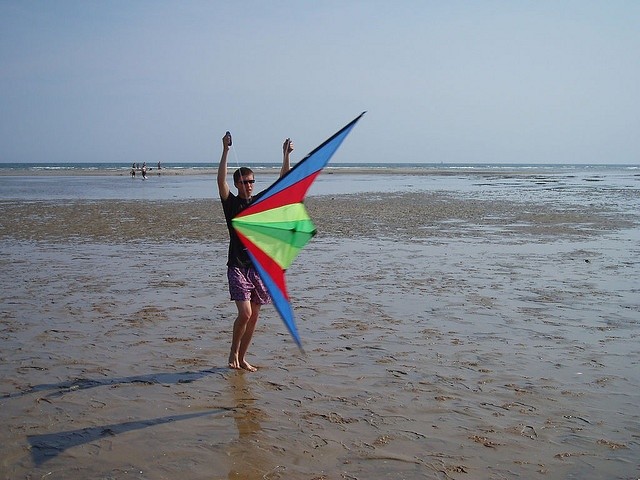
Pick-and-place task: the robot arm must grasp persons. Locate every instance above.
[217,134,294,375]
[128,160,163,180]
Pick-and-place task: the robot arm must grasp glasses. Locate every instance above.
[237,180,255,184]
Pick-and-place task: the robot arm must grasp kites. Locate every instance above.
[229,111,369,354]
